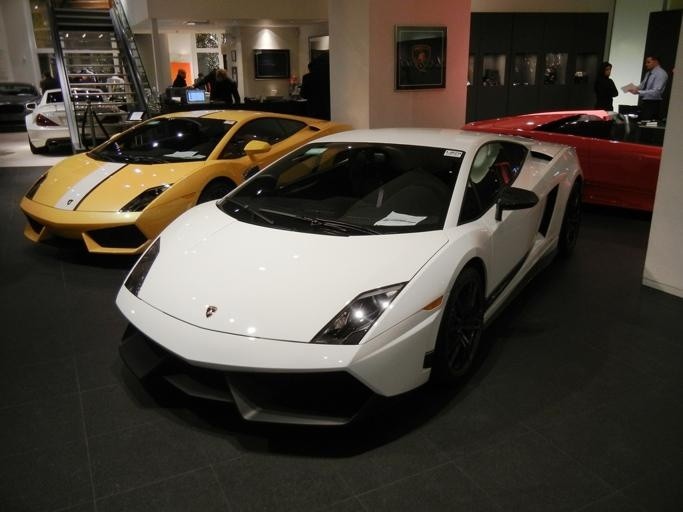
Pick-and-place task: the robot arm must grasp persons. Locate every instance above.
[298,61,312,100]
[592,61,619,112]
[629,53,669,108]
[39,67,241,105]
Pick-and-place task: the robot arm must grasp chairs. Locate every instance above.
[316,143,407,207]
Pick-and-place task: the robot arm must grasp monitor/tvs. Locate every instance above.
[639,98,664,120]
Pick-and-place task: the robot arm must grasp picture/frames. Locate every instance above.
[392,24,447,91]
[255,48,291,79]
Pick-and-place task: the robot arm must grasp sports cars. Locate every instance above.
[117,127,587,428]
[18,109,348,256]
[0,79,133,155]
[460,107,667,215]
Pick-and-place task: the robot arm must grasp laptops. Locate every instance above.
[126,110,145,121]
[186,89,206,104]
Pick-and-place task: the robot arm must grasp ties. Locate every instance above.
[641,72,652,91]
